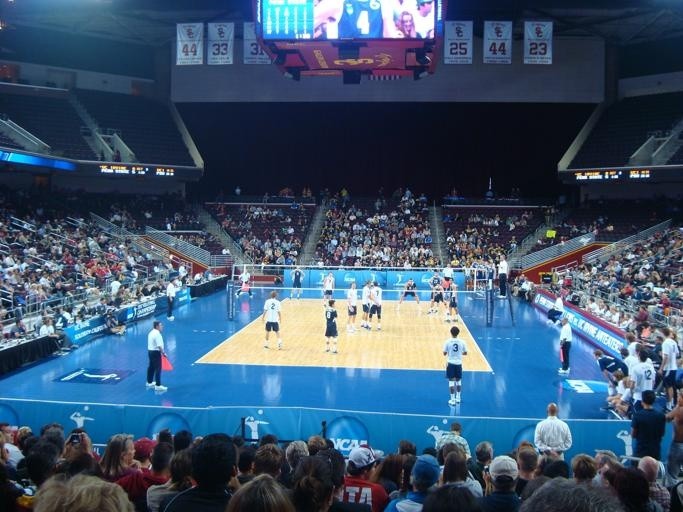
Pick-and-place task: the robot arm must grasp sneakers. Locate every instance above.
[144,380,156,387]
[447,398,461,406]
[154,385,168,391]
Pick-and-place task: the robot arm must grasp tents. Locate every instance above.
[523,21,553,66]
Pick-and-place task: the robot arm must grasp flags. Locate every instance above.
[482,21,514,66]
[206,22,235,65]
[442,21,473,66]
[241,21,273,66]
[174,22,203,66]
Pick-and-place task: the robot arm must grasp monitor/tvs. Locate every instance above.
[542,274,551,282]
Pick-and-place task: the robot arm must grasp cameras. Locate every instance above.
[484,465,488,472]
[70,433,80,443]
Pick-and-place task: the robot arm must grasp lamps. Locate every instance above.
[264,42,310,82]
[403,38,435,82]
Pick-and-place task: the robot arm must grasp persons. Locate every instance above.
[325,300,339,353]
[631,391,666,466]
[361,281,374,329]
[1,422,683,512]
[558,317,572,373]
[428,264,460,322]
[274,273,281,286]
[323,273,335,307]
[315,187,441,271]
[214,186,312,269]
[290,267,305,298]
[369,281,382,329]
[667,390,683,478]
[511,275,532,300]
[313,0,434,39]
[443,328,467,404]
[262,291,282,350]
[1,183,232,338]
[444,214,529,283]
[236,271,253,298]
[400,279,419,305]
[593,328,682,420]
[496,255,510,298]
[145,321,168,390]
[534,403,572,464]
[347,283,359,332]
[537,195,683,337]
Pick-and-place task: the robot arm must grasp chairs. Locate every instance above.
[0,180,682,311]
[558,40,682,169]
[0,22,195,166]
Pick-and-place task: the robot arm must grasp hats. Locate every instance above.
[345,446,384,469]
[413,454,441,484]
[487,455,519,481]
